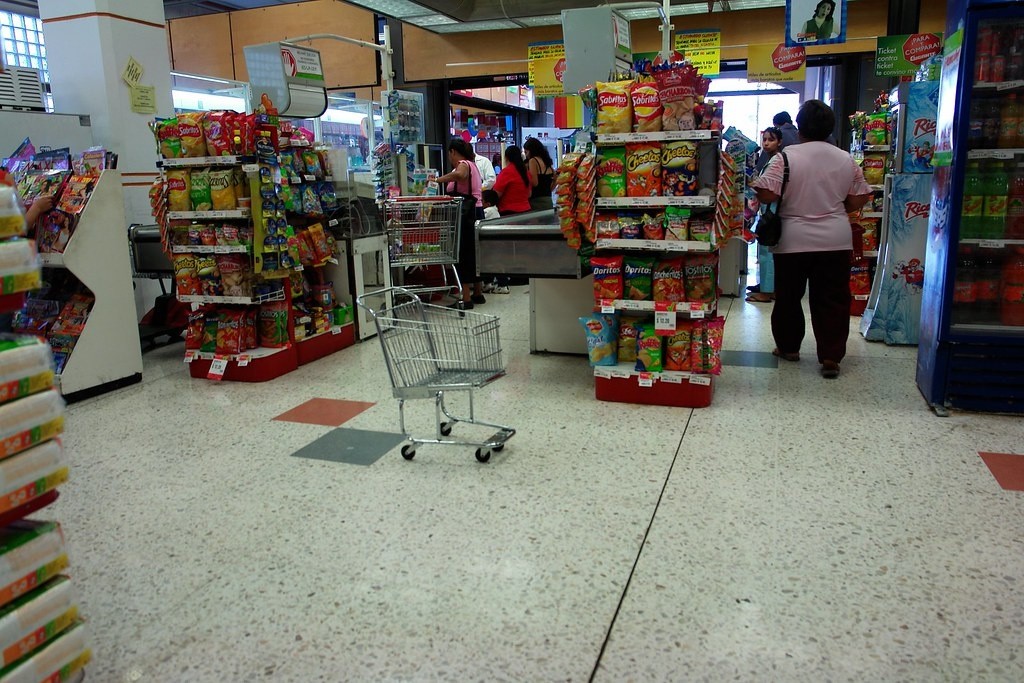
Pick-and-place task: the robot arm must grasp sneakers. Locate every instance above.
[446,300,473,310]
[471,295,485,304]
[482,282,494,292]
[494,286,510,294]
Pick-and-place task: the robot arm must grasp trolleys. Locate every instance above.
[355,286,518,463]
[382,195,465,325]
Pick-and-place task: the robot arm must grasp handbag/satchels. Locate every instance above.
[444,160,477,215]
[755,151,789,246]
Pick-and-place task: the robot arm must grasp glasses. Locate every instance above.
[767,126,780,131]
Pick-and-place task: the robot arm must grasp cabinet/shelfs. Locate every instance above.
[156,153,297,384]
[850,144,890,300]
[589,128,716,409]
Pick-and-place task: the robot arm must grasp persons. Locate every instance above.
[524,138,553,211]
[494,145,533,286]
[36,179,51,195]
[469,143,496,191]
[80,176,96,206]
[755,112,800,171]
[746,127,783,302]
[428,140,486,310]
[492,153,502,176]
[51,213,74,251]
[801,0,836,40]
[748,99,874,379]
[481,190,510,294]
[0,166,55,333]
[45,181,60,195]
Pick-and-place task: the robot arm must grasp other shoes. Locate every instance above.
[821,364,839,378]
[772,348,800,361]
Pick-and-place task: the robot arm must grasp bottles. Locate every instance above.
[960,162,1024,240]
[952,246,1024,326]
[968,92,1024,148]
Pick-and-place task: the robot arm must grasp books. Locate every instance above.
[0,137,106,373]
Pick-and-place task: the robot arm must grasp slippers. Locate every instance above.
[745,296,772,303]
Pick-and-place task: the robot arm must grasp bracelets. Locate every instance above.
[434,178,437,182]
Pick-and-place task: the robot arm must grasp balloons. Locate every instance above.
[478,130,486,138]
[462,130,472,143]
[468,120,478,137]
